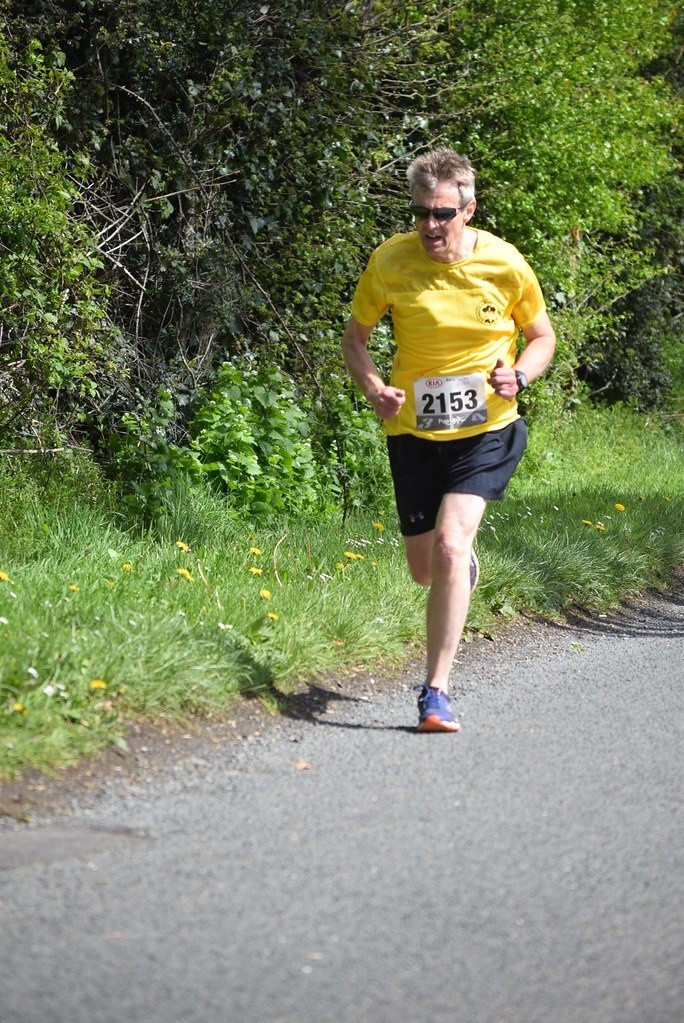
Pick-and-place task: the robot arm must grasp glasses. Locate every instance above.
[408,195,470,221]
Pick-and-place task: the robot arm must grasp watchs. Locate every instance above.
[515,370,528,396]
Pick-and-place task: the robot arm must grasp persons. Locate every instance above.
[340,148,557,733]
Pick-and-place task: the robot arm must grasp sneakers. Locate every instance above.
[414,683,460,732]
[469,547,479,593]
